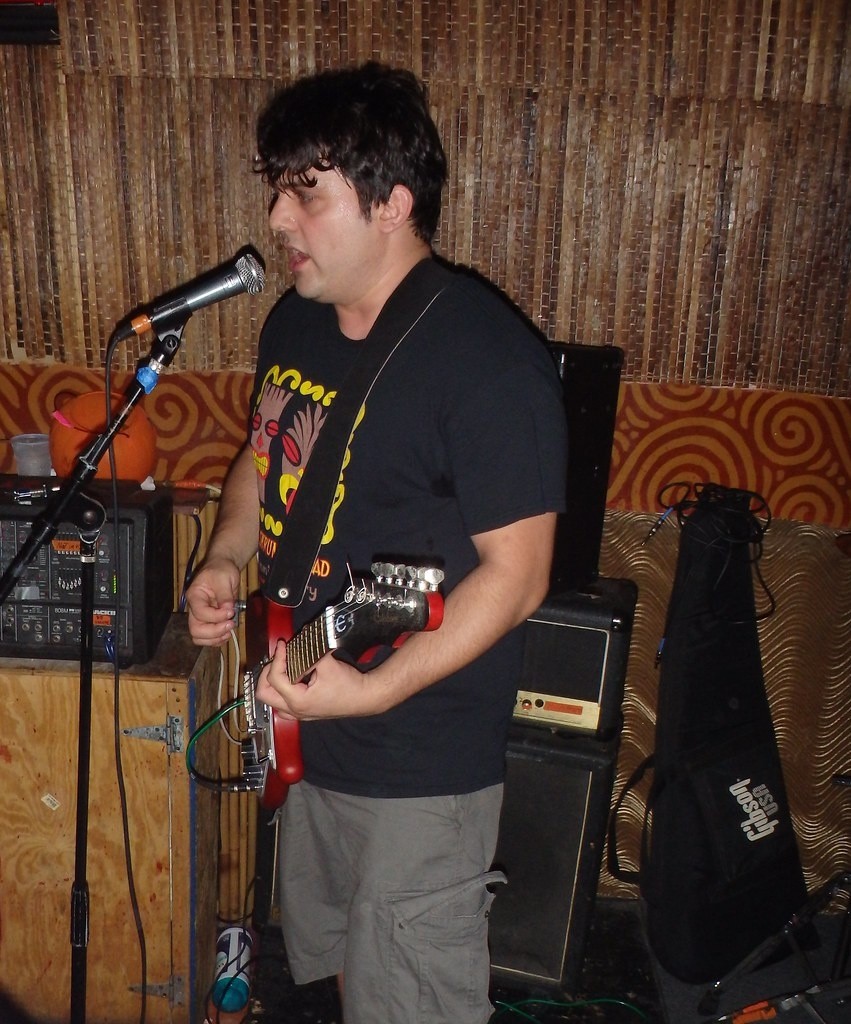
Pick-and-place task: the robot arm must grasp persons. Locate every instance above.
[181,60,564,1024]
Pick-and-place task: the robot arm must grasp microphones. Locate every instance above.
[116,251,267,343]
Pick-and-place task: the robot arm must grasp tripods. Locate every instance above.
[696,871,851,1018]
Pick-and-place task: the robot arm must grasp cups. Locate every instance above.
[10,434,52,477]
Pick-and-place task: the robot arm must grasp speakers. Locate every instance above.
[541,340,624,586]
[256,718,616,1003]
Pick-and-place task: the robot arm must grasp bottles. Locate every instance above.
[212,927,254,1012]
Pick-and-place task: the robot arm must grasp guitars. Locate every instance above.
[240,559,446,810]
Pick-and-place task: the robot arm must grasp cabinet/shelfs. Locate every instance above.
[0,608,225,1024]
